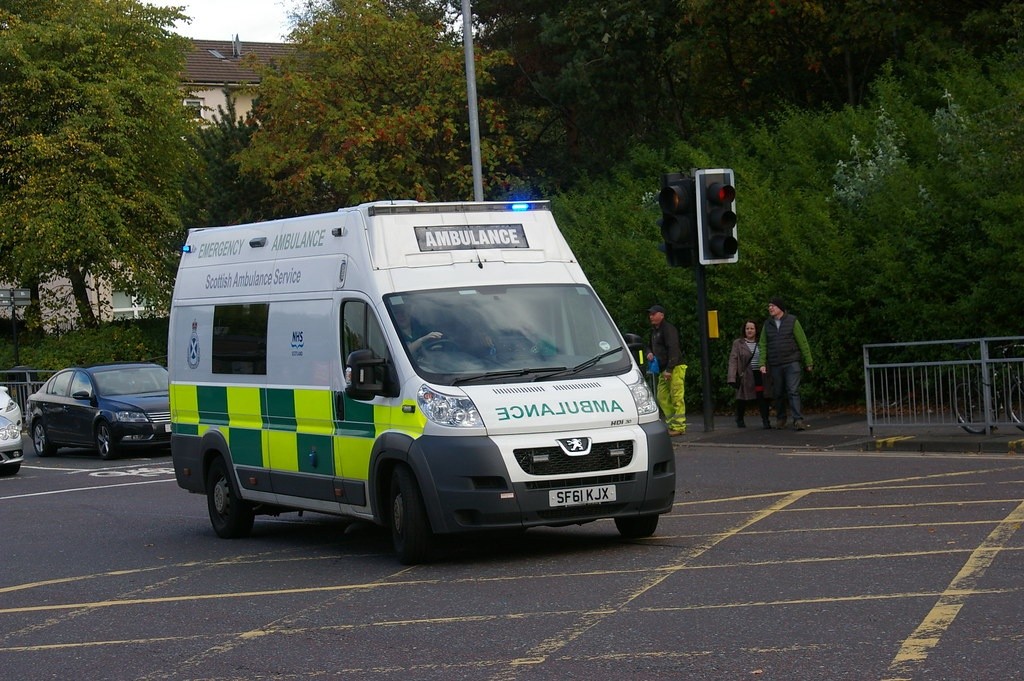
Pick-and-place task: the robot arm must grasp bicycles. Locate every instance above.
[951,343,1024,435]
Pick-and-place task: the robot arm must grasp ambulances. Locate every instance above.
[164,194,678,566]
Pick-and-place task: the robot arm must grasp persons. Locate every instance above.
[758,298,813,431]
[646,305,689,436]
[727,320,771,429]
[385,296,496,394]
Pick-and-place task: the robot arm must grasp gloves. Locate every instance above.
[730,382,736,388]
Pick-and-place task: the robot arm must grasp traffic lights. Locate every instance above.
[706,309,723,342]
[691,167,742,266]
[651,169,686,254]
[657,178,701,267]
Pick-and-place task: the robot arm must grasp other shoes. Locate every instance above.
[794,419,805,431]
[776,419,787,430]
[668,429,686,437]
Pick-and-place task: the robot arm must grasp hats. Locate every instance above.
[769,298,785,312]
[646,305,664,313]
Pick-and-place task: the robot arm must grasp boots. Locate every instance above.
[762,416,771,429]
[736,410,745,427]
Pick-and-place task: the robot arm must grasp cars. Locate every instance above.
[0,415,25,476]
[0,385,23,435]
[24,361,172,461]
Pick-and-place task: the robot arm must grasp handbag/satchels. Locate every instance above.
[732,372,741,390]
[647,355,659,376]
